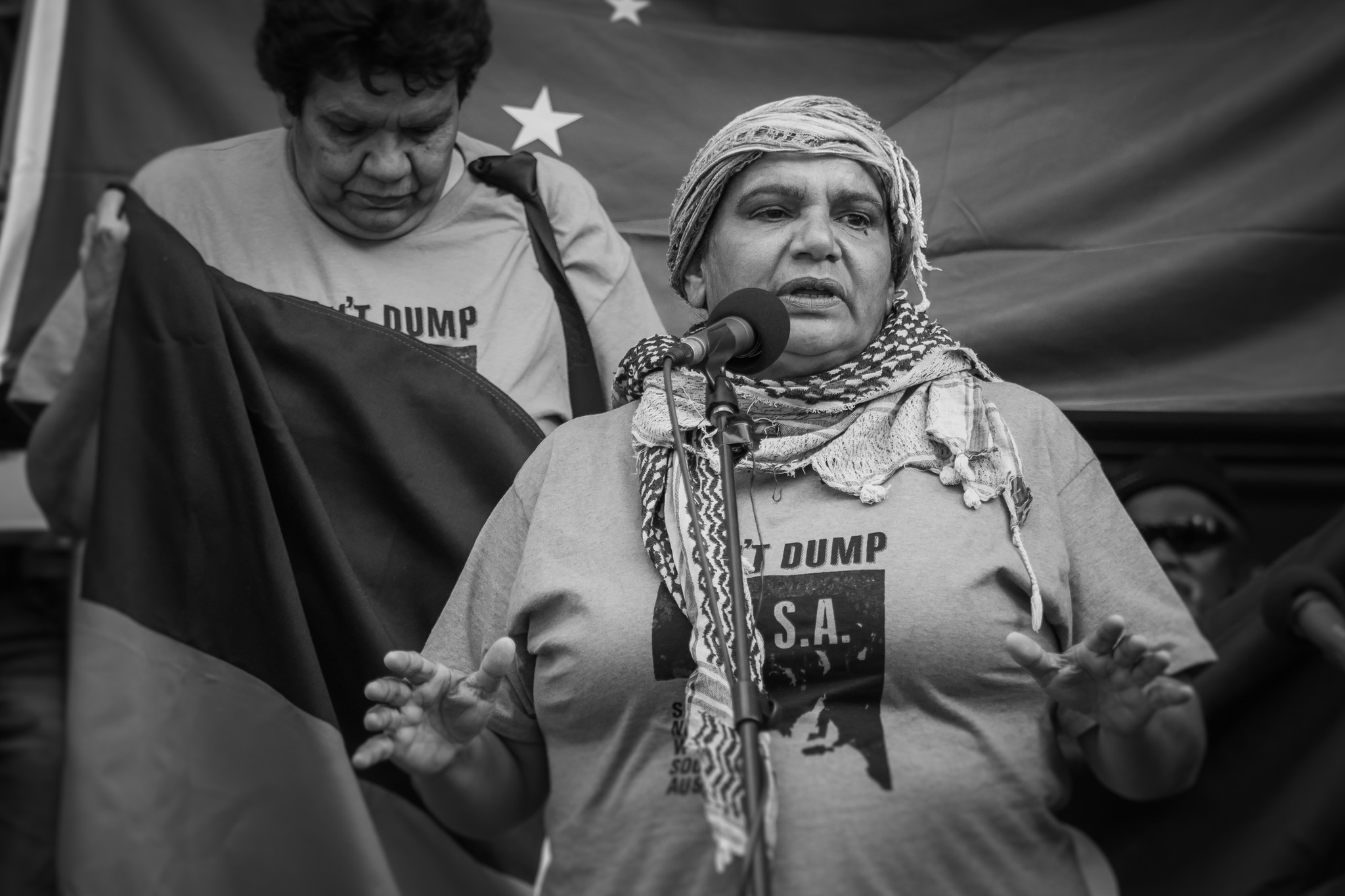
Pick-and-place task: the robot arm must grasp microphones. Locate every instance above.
[664,286,793,386]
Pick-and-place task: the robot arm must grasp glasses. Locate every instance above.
[1130,517,1238,555]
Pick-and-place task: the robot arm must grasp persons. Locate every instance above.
[5,0,677,540]
[353,88,1217,896]
[1112,483,1250,623]
